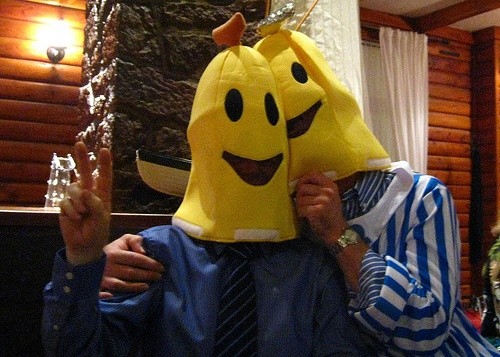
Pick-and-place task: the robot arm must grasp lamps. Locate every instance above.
[37,17,73,62]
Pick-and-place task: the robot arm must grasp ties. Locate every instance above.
[215,244,260,356]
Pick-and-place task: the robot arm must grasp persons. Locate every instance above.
[97,0,499,357]
[31,10,374,357]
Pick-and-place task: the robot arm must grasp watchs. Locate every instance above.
[328,226,360,259]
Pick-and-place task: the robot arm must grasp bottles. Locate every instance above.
[44,158,70,211]
